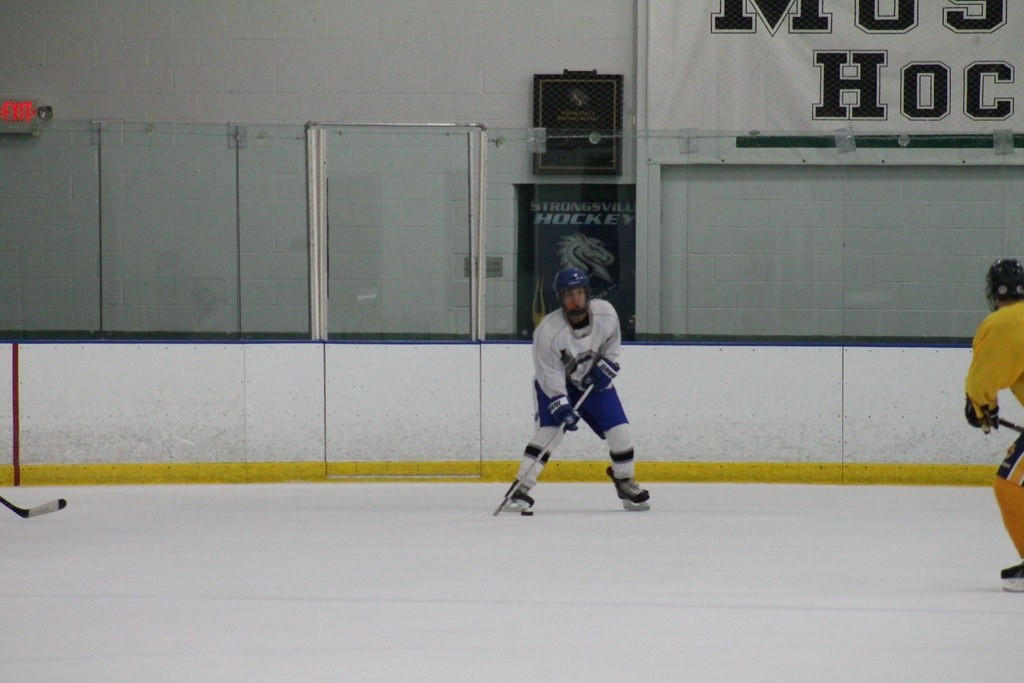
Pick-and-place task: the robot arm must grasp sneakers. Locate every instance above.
[1001,561,1024,591]
[606,466,650,511]
[499,479,534,512]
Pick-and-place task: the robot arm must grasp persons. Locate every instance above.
[964,259,1024,590]
[506,265,650,511]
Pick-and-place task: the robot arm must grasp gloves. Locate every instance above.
[582,356,619,391]
[965,398,999,434]
[546,394,580,431]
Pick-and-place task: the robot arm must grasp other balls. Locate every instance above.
[520,511,533,516]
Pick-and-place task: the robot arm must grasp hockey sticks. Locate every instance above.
[1,496,67,518]
[492,380,596,517]
[998,418,1024,432]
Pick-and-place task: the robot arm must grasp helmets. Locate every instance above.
[986,259,1024,312]
[553,267,590,315]
